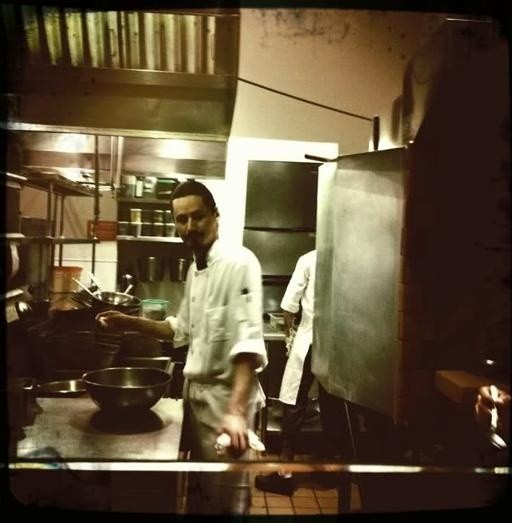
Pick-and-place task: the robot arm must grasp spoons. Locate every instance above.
[489,385,506,447]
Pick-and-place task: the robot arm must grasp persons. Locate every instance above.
[254,251,349,498]
[95,180,269,518]
[474,382,512,469]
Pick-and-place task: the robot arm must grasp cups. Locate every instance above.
[143,222,151,236]
[165,210,171,223]
[154,210,164,223]
[131,209,142,223]
[166,223,176,236]
[118,222,129,234]
[131,222,142,236]
[154,223,164,236]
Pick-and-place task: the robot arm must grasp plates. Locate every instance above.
[91,291,142,322]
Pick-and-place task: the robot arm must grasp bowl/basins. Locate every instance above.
[38,380,88,393]
[82,368,173,410]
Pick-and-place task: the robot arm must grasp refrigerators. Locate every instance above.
[243,160,325,317]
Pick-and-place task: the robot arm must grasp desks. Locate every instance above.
[255,311,331,456]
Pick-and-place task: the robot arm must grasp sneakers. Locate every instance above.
[254,474,294,496]
